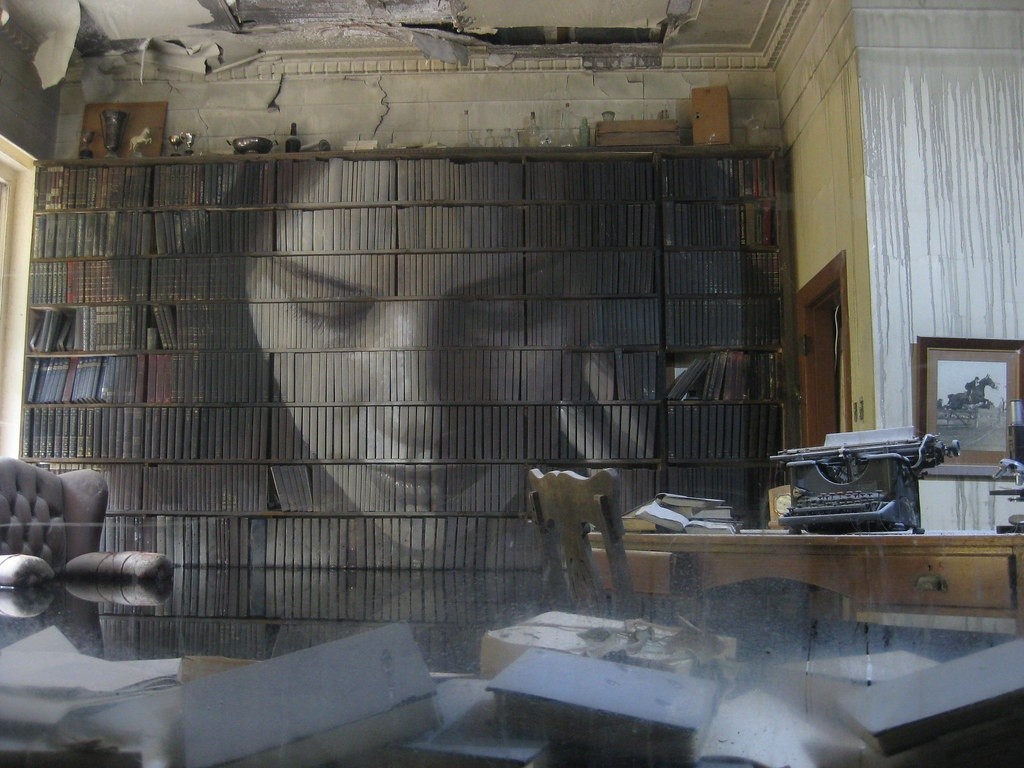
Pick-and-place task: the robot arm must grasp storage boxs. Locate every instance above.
[595,120,679,145]
[692,86,729,146]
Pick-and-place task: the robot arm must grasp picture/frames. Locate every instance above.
[917,336,1024,477]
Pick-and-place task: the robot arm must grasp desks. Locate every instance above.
[588,532,1024,638]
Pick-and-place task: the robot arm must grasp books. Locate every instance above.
[0,638,1024,768]
[19,157,788,534]
[96,517,561,667]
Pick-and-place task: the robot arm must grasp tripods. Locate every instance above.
[770,435,961,470]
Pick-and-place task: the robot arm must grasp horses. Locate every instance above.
[947,374,998,426]
[129,126,153,153]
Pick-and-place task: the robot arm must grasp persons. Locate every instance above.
[239,157,668,569]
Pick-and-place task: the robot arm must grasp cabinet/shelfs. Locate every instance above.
[20,145,791,517]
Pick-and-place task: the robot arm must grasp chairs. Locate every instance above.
[528,467,635,618]
[0,459,173,661]
